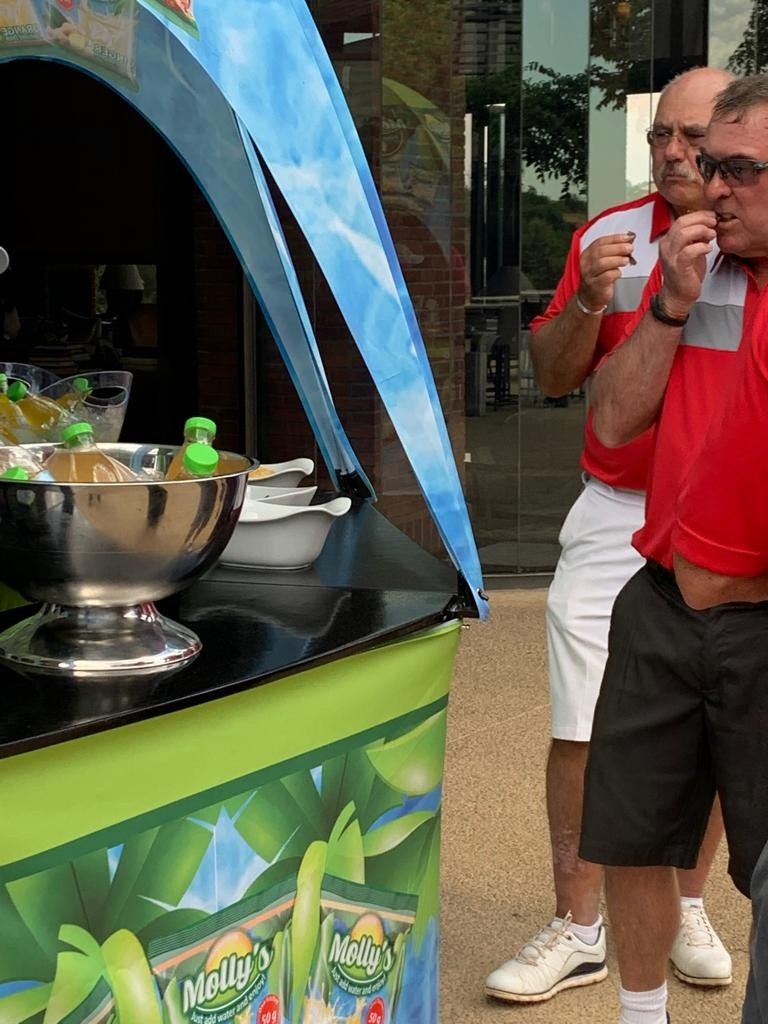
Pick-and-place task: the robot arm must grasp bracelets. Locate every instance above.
[648,293,690,325]
[574,293,608,315]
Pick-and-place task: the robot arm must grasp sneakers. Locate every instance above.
[485,910,608,1003]
[668,903,732,987]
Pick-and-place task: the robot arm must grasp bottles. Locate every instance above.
[0,372,220,485]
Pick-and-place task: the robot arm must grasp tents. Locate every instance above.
[0,0,497,622]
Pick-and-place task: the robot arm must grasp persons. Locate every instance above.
[578,71,767,1023]
[482,65,743,1001]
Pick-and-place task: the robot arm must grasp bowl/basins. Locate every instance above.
[247,458,315,487]
[244,484,318,506]
[0,443,260,670]
[217,497,351,569]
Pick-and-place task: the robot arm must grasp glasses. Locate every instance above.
[694,152,768,188]
[644,126,708,148]
